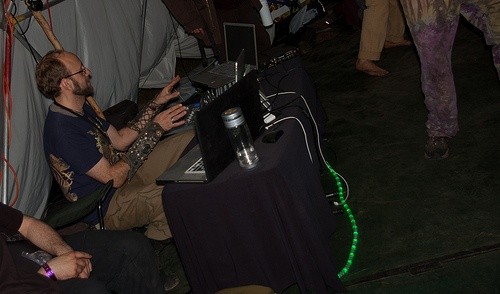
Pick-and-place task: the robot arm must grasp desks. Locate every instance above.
[162,55,348,294]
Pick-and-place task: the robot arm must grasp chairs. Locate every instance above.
[42,98,140,231]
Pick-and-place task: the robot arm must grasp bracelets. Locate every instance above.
[42,263,57,281]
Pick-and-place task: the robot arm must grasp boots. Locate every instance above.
[148,239,180,292]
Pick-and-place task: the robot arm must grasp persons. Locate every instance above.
[161,0,270,69]
[0,202,163,294]
[36,50,198,250]
[356,0,500,160]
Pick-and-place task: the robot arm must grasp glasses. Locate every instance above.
[62,63,86,79]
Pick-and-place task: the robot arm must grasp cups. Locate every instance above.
[222,106,260,169]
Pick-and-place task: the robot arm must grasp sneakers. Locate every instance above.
[424,135,452,160]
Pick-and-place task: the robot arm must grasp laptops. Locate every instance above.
[191,22,258,89]
[213,49,247,99]
[155,69,264,183]
